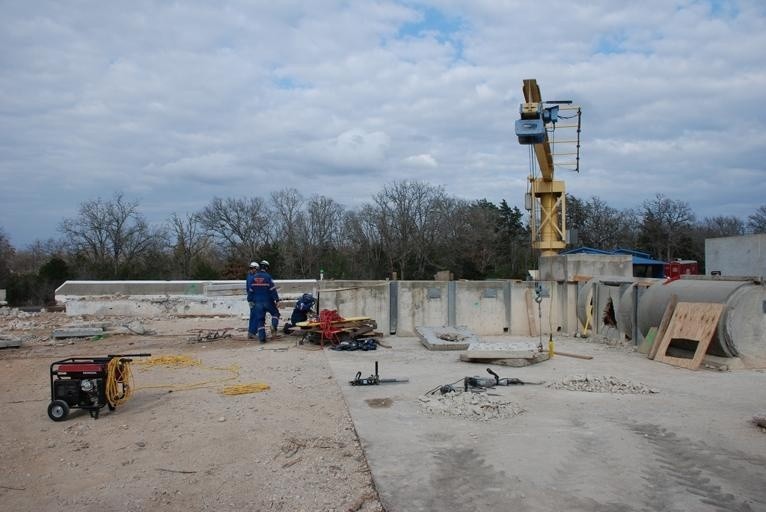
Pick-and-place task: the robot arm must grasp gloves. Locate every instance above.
[249,302,255,309]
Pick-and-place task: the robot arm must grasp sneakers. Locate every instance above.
[248,337,256,341]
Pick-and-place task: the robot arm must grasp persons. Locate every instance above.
[283,293,315,334]
[246,262,260,340]
[247,259,280,345]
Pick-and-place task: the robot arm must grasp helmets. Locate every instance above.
[260,260,270,270]
[250,262,260,270]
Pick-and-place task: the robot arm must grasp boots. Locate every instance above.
[257,327,267,344]
[271,317,278,334]
